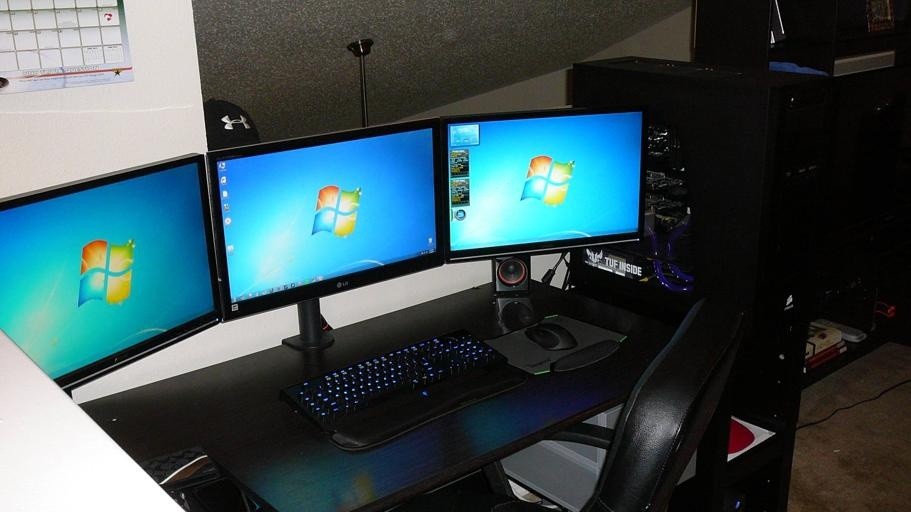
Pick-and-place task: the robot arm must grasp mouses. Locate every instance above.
[524,322,578,351]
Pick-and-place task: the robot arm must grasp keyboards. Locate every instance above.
[280,330,529,452]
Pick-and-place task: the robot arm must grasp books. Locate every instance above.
[802,323,843,358]
[800,337,848,372]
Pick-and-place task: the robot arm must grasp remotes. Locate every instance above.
[817,318,867,343]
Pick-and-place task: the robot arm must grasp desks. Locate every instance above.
[71,269,872,508]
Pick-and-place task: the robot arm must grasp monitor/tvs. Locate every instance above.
[206,116,444,352]
[0,154,223,400]
[440,101,650,266]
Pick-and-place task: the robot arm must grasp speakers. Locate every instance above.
[492,252,531,294]
[495,297,537,334]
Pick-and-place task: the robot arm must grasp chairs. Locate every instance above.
[481,296,786,507]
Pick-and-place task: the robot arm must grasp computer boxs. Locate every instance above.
[571,55,841,328]
